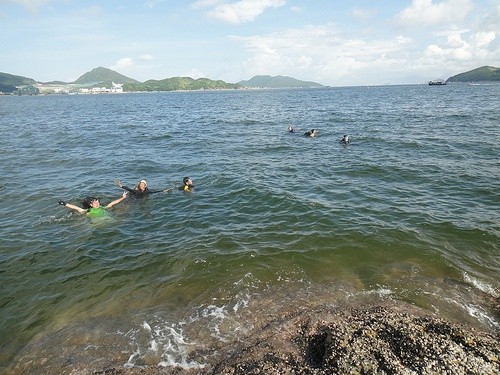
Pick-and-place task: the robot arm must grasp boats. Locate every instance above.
[428,80,448,85]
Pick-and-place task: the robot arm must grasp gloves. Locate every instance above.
[58,199,67,206]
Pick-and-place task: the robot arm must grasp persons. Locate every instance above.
[307,129,317,138]
[180,176,195,193]
[116,180,162,197]
[341,134,351,144]
[59,190,130,219]
[288,126,295,134]
